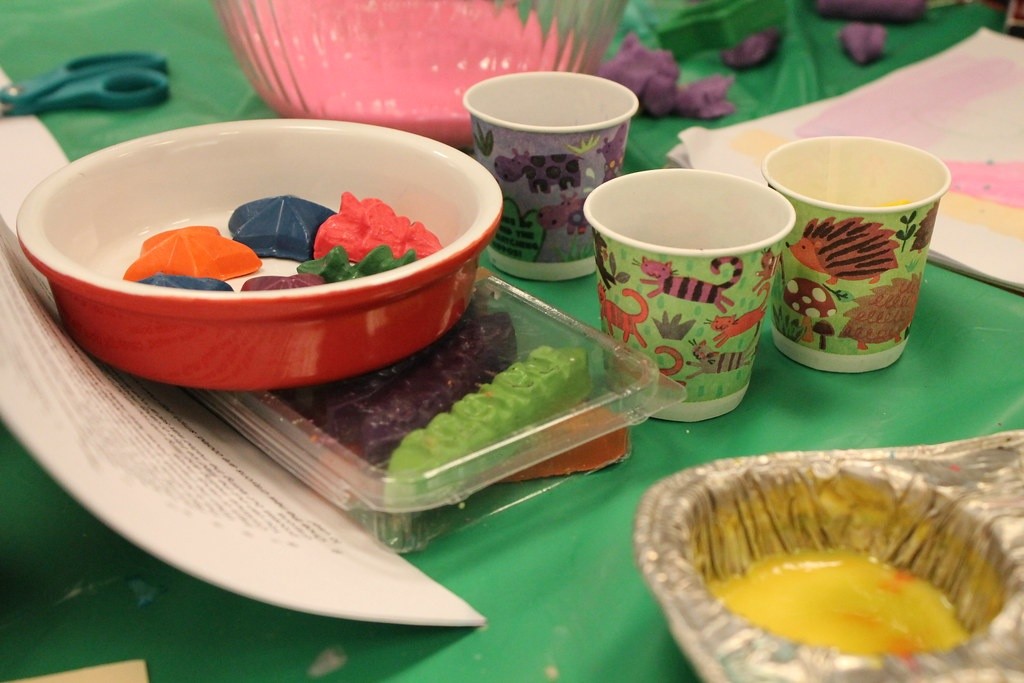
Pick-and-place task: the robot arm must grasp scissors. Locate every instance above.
[0,48,170,113]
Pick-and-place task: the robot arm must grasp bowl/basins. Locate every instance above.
[14,116,505,391]
[210,0,630,152]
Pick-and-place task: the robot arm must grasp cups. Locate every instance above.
[581,167,797,423]
[460,70,640,282]
[759,133,953,375]
